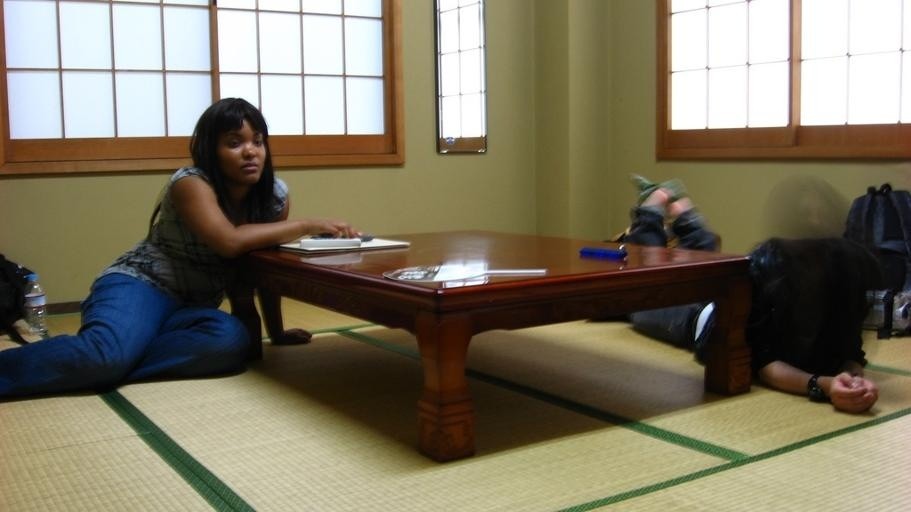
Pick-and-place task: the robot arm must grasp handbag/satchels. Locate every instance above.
[0,254,35,334]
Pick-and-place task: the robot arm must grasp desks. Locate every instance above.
[241,227,759,464]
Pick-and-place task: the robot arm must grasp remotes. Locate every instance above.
[310,233,374,242]
[301,238,361,248]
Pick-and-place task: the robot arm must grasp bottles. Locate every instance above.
[26,273,48,336]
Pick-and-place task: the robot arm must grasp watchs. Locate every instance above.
[808,375,825,402]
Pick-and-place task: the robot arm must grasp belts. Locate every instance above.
[686,301,712,350]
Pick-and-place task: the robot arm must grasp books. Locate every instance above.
[278,240,409,255]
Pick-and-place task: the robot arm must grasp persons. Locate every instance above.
[625,176,879,412]
[0,97,357,402]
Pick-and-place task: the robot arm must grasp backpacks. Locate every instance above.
[842,184,911,291]
